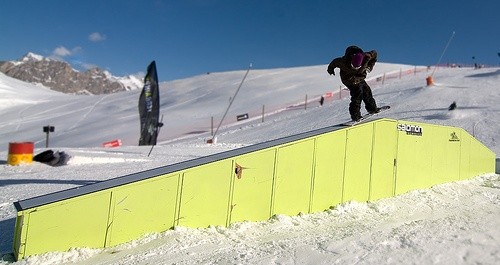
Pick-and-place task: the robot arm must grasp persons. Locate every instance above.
[327,45,380,121]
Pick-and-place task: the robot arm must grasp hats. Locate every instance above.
[352,53,363,66]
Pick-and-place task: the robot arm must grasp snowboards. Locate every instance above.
[342,106,391,126]
